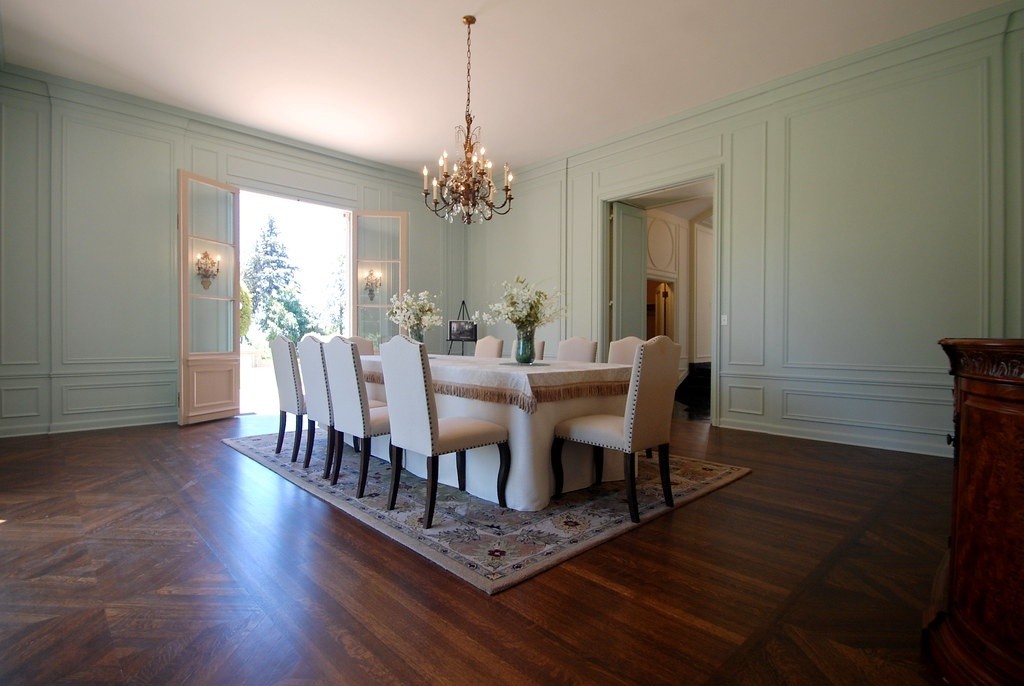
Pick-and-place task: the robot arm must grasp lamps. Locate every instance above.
[420,15,514,226]
[194,249,220,291]
[362,269,382,301]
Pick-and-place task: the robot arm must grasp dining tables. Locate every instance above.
[340,352,637,513]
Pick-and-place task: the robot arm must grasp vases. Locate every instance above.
[409,330,423,344]
[515,331,536,366]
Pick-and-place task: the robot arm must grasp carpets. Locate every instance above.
[219,431,753,599]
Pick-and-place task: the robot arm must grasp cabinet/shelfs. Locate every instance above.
[921,338,1024,685]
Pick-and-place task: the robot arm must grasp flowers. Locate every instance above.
[482,275,567,334]
[387,288,444,333]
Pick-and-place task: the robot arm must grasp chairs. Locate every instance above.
[296,335,385,478]
[608,336,646,365]
[269,335,308,462]
[474,335,503,358]
[378,334,511,530]
[511,339,545,360]
[550,333,683,524]
[322,335,391,498]
[557,337,597,364]
